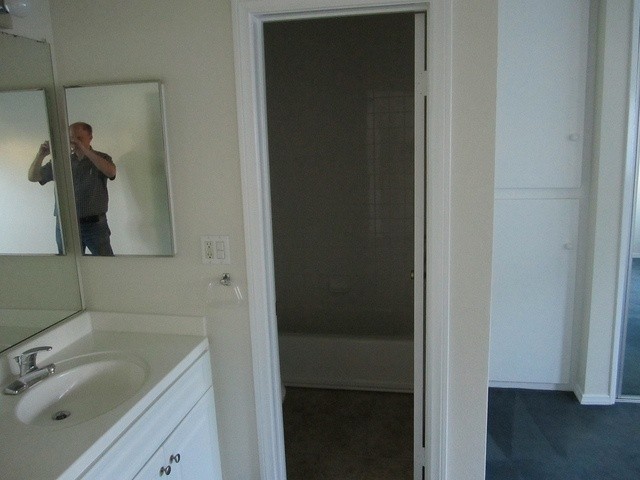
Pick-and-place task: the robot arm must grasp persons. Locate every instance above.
[28,123,116,255]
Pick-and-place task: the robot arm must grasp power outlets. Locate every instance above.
[200,235,232,265]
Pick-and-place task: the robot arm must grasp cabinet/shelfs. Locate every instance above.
[135,385,223,480]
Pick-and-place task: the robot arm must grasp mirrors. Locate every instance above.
[0,86,82,355]
[63,80,178,258]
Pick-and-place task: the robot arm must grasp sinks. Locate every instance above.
[15,354,150,429]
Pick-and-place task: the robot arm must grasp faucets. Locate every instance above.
[4,345,57,397]
